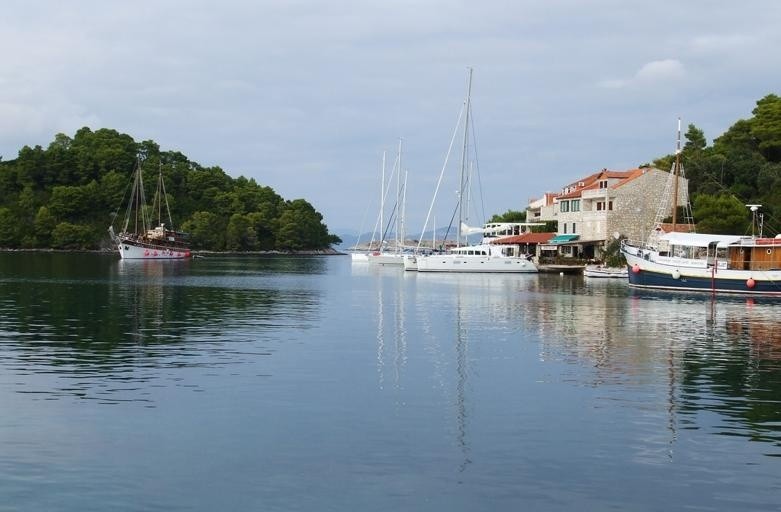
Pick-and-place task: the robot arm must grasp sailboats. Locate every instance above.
[350,66,471,271]
[416,67,539,273]
[108,150,193,260]
[585,118,695,278]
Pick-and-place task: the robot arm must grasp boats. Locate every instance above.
[621,228,780,302]
